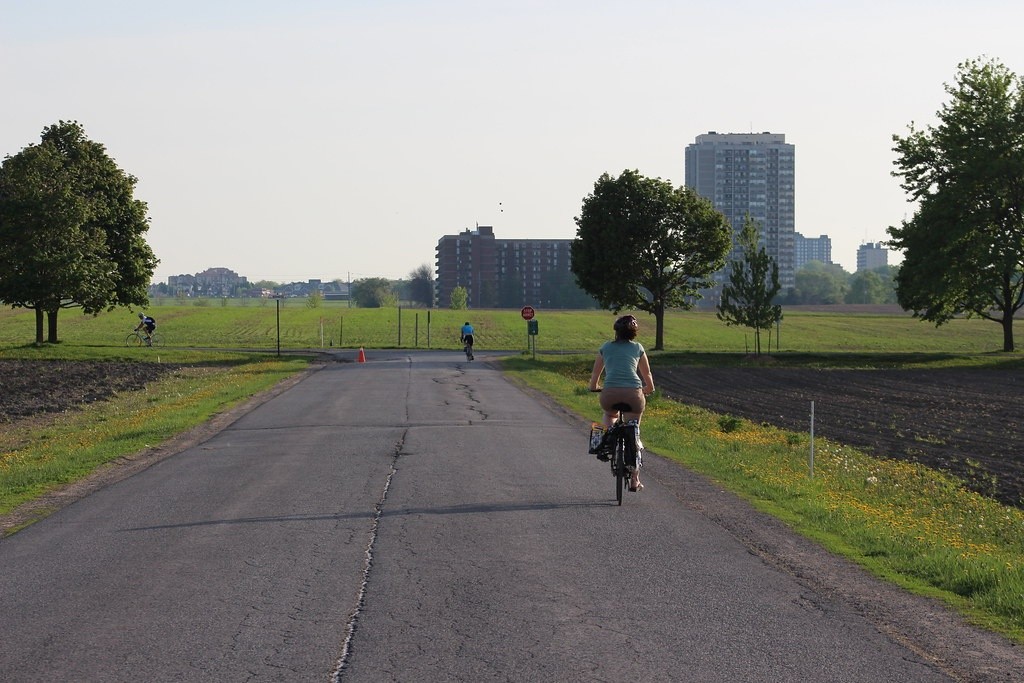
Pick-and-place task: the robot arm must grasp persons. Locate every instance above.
[134,312,155,347]
[461,322,474,360]
[590,315,654,492]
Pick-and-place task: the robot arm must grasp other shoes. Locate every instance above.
[464,347,467,352]
[145,336,151,339]
[596,454,610,462]
[630,478,644,489]
[472,355,474,360]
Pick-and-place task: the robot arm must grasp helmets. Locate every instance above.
[613,314,638,331]
[138,312,143,316]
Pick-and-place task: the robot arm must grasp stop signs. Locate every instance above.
[521,306,535,319]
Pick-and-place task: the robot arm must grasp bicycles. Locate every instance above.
[461,338,473,362]
[126,329,166,348]
[589,388,654,506]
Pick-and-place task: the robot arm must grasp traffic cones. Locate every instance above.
[358,346,366,363]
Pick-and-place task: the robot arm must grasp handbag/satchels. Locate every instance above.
[588,421,613,454]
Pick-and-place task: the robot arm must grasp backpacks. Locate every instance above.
[143,316,155,324]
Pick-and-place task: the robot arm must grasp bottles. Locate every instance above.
[632,420,639,442]
